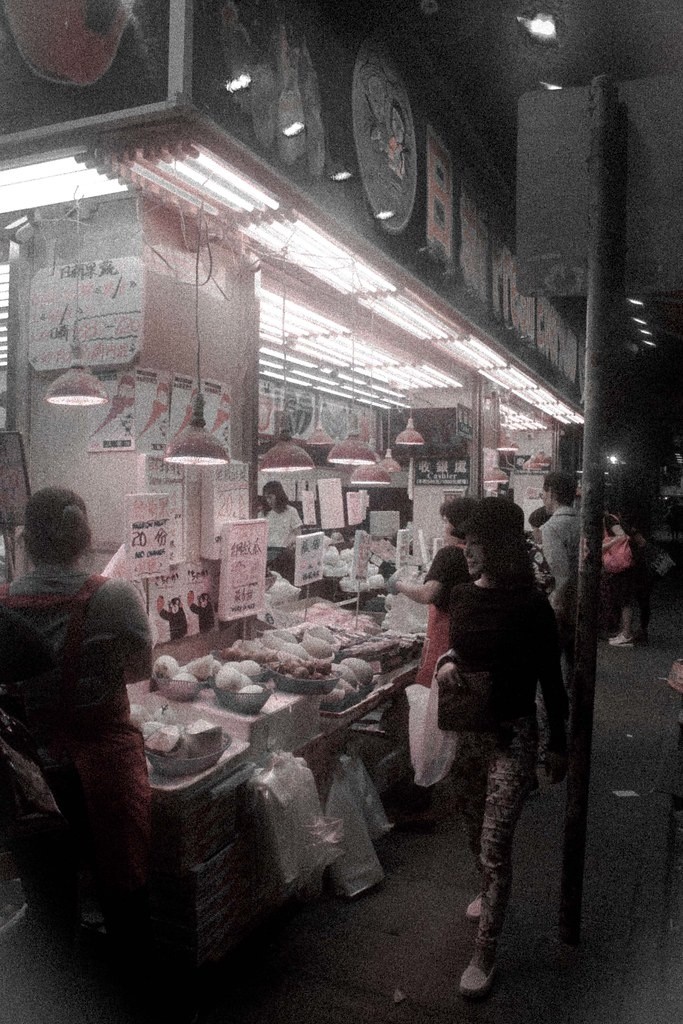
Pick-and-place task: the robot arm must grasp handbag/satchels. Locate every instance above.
[438,677,493,732]
[644,544,676,579]
[600,513,634,574]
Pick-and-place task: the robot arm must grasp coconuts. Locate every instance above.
[143,718,223,761]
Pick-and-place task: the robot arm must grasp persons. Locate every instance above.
[528,505,561,599]
[1,487,158,1012]
[255,480,304,563]
[613,493,651,646]
[377,499,489,838]
[603,496,636,648]
[433,493,570,997]
[534,470,580,730]
[651,497,683,542]
[2,600,60,695]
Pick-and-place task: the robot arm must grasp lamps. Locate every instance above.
[483,447,509,483]
[306,315,333,445]
[261,242,314,472]
[163,199,229,468]
[351,312,391,484]
[395,345,425,446]
[327,259,377,466]
[220,5,252,92]
[379,382,401,473]
[45,190,108,407]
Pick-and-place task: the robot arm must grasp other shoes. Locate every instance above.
[466,891,482,921]
[609,633,635,648]
[460,962,497,996]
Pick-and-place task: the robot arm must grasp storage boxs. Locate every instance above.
[140,760,257,971]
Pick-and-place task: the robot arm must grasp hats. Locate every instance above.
[450,496,532,550]
